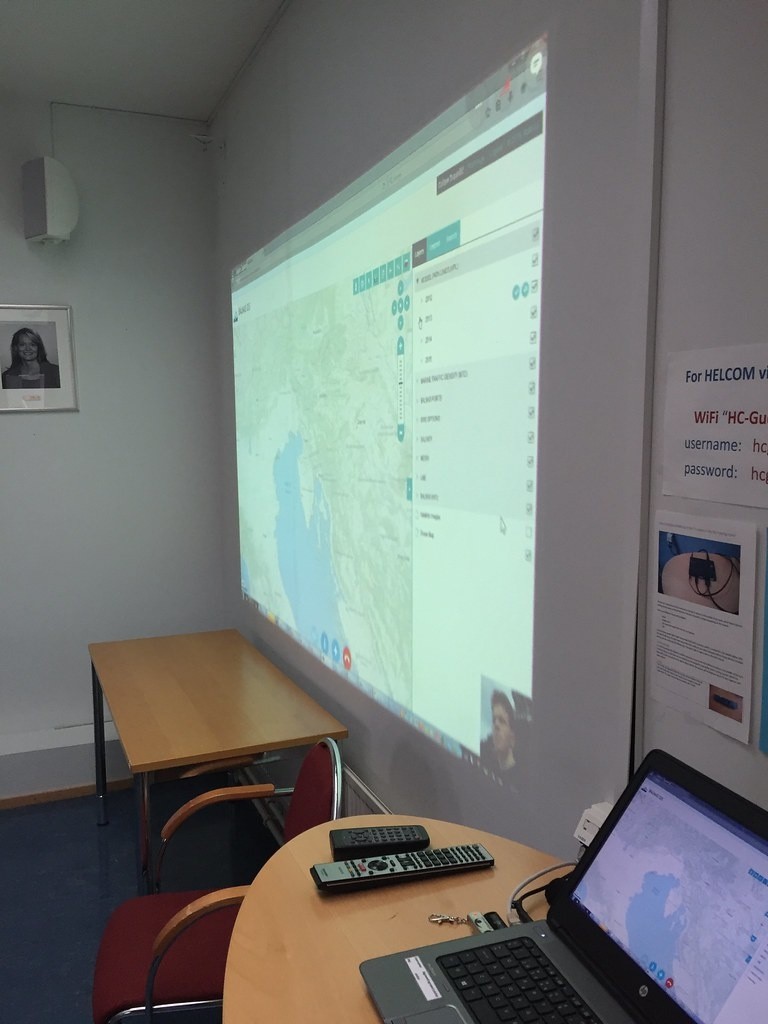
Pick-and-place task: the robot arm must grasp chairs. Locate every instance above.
[91,738,343,1024]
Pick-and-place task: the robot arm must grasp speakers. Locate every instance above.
[20,156,79,241]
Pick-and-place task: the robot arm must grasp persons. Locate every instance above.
[2,328,61,389]
[483,690,522,790]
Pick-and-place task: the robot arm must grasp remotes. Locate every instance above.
[310,843,494,891]
[328,825,430,862]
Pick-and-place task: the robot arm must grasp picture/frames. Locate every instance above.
[0,304,79,413]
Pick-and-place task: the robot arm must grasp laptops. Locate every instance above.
[359,748,768,1024]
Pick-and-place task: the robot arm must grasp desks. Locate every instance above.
[222,814,573,1024]
[88,631,348,894]
